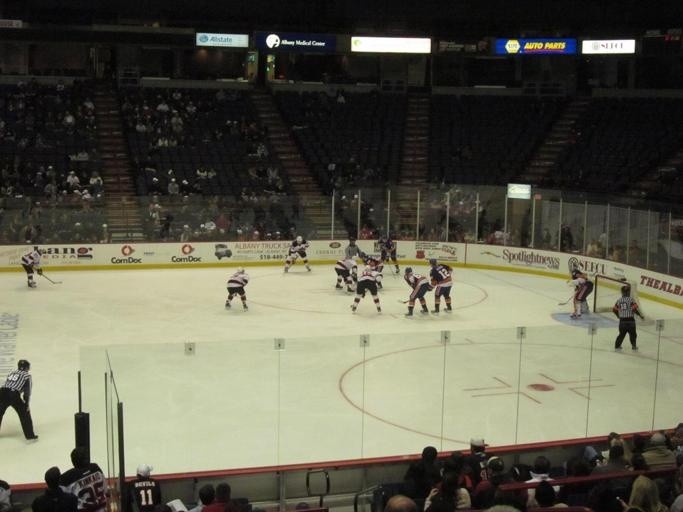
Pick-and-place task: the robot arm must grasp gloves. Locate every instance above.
[37,268,44,275]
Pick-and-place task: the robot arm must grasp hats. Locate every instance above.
[470,436,488,446]
[481,456,504,470]
[137,464,152,476]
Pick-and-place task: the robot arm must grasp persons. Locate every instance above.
[335,236,454,318]
[0,360,38,440]
[328,158,642,266]
[2,79,113,243]
[567,270,593,319]
[304,90,346,116]
[21,248,44,288]
[123,91,298,242]
[386,422,683,512]
[285,236,311,272]
[225,266,249,313]
[612,285,645,351]
[1,448,311,509]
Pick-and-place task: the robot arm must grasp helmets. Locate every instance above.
[18,359,30,370]
[39,248,46,256]
[237,267,245,274]
[349,237,356,245]
[430,258,436,267]
[405,267,412,274]
[297,236,302,246]
[382,234,387,242]
[621,286,630,296]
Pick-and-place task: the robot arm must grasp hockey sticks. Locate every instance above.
[386,260,399,277]
[396,298,408,305]
[282,254,298,272]
[32,265,59,283]
[557,292,576,305]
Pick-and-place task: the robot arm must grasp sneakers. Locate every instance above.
[405,308,451,316]
[336,283,354,293]
[396,267,400,274]
[24,434,37,441]
[307,267,311,272]
[378,306,381,313]
[285,267,288,271]
[28,281,35,288]
[226,302,231,307]
[244,305,249,309]
[351,304,357,311]
